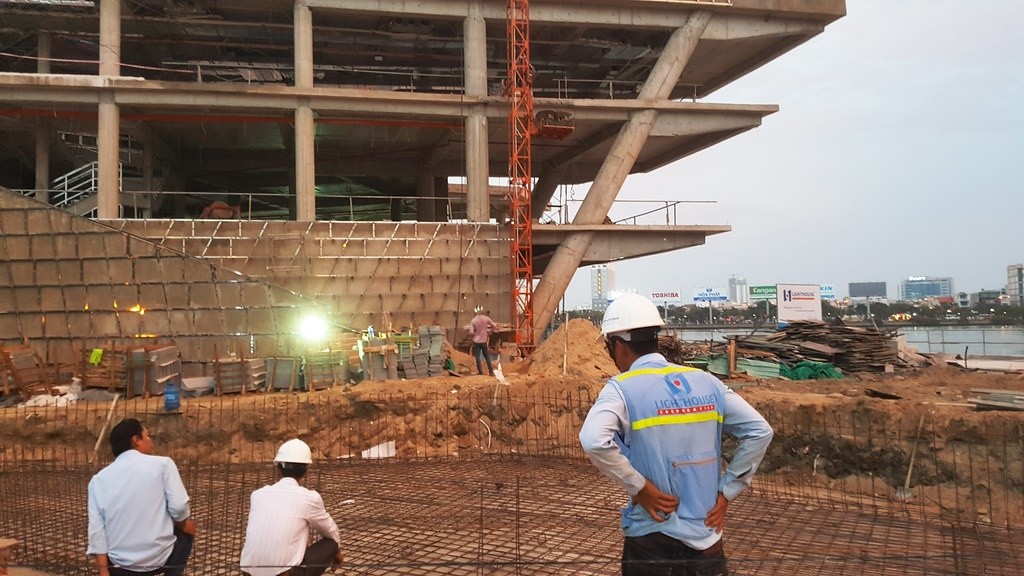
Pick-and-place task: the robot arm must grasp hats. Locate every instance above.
[473,304,484,315]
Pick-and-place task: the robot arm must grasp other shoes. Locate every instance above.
[490,372,495,376]
[476,372,482,375]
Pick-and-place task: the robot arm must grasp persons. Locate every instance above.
[86,417,196,576]
[469,304,497,377]
[239,437,343,576]
[579,293,775,576]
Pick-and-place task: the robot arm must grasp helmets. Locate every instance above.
[594,293,664,343]
[272,438,313,464]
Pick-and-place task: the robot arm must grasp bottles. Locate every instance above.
[368,326,374,339]
[164,384,179,410]
